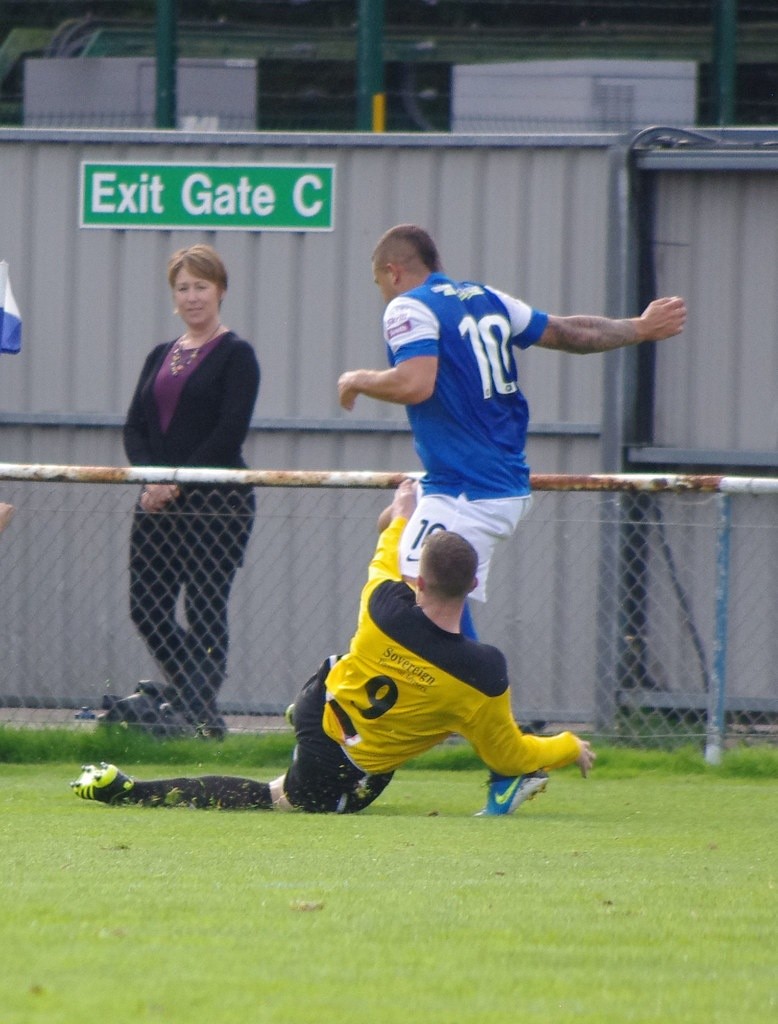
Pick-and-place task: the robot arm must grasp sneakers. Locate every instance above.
[285,703,296,725]
[475,766,549,819]
[69,761,133,804]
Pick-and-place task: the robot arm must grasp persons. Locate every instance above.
[124,245,261,739]
[70,479,596,812]
[337,225,687,815]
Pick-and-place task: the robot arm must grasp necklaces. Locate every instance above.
[170,322,222,377]
